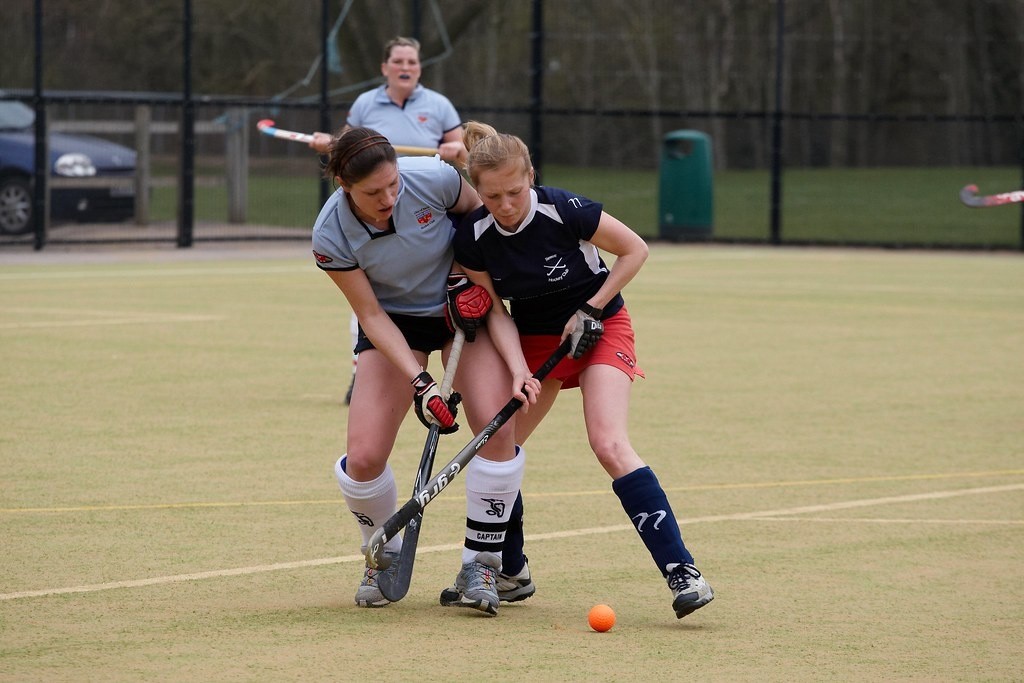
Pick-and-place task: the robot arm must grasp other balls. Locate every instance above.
[588,605,614,633]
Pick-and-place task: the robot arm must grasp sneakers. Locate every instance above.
[452,550,502,617]
[353,545,403,609]
[438,553,536,607]
[663,559,716,619]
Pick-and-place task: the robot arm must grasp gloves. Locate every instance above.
[409,371,462,435]
[443,271,494,344]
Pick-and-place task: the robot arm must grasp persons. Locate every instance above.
[311,38,716,621]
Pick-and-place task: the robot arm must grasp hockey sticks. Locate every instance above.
[962,182,1024,208]
[375,315,461,602]
[365,334,574,569]
[257,119,440,156]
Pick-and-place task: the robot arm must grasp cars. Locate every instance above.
[0,99,139,237]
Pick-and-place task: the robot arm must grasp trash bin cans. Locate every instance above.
[657,128,714,243]
